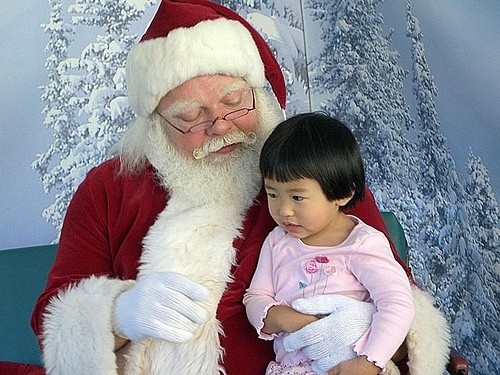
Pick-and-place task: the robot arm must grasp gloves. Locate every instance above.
[110,274,209,344]
[282,295,377,375]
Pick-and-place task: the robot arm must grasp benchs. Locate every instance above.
[0,211,467,375]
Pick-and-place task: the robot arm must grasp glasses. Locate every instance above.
[154,88,254,136]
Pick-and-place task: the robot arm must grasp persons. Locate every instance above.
[23,0,449,375]
[241,111,413,375]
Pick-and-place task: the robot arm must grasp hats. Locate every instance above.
[127,0,287,128]
